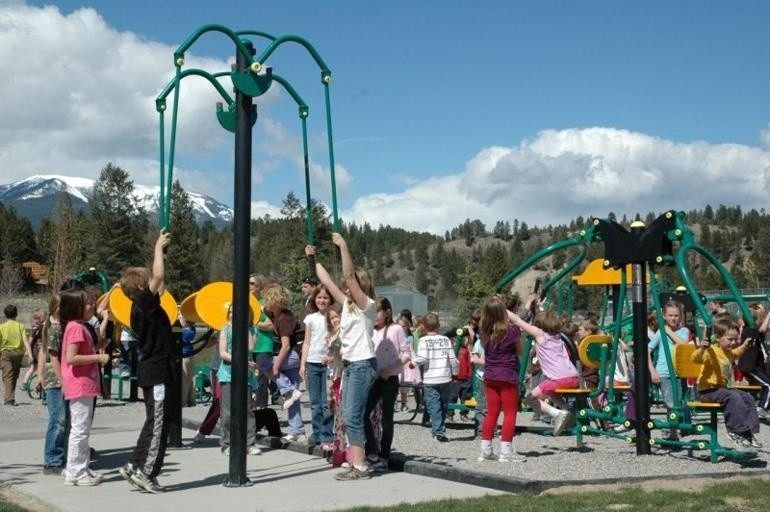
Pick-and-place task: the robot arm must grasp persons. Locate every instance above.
[0,228,770,495]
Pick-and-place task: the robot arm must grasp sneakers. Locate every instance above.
[480,451,527,462]
[42,464,65,476]
[336,456,389,480]
[282,389,302,410]
[119,462,166,493]
[64,474,97,486]
[247,446,262,455]
[281,434,306,443]
[727,432,762,448]
[166,442,185,449]
[552,410,570,436]
